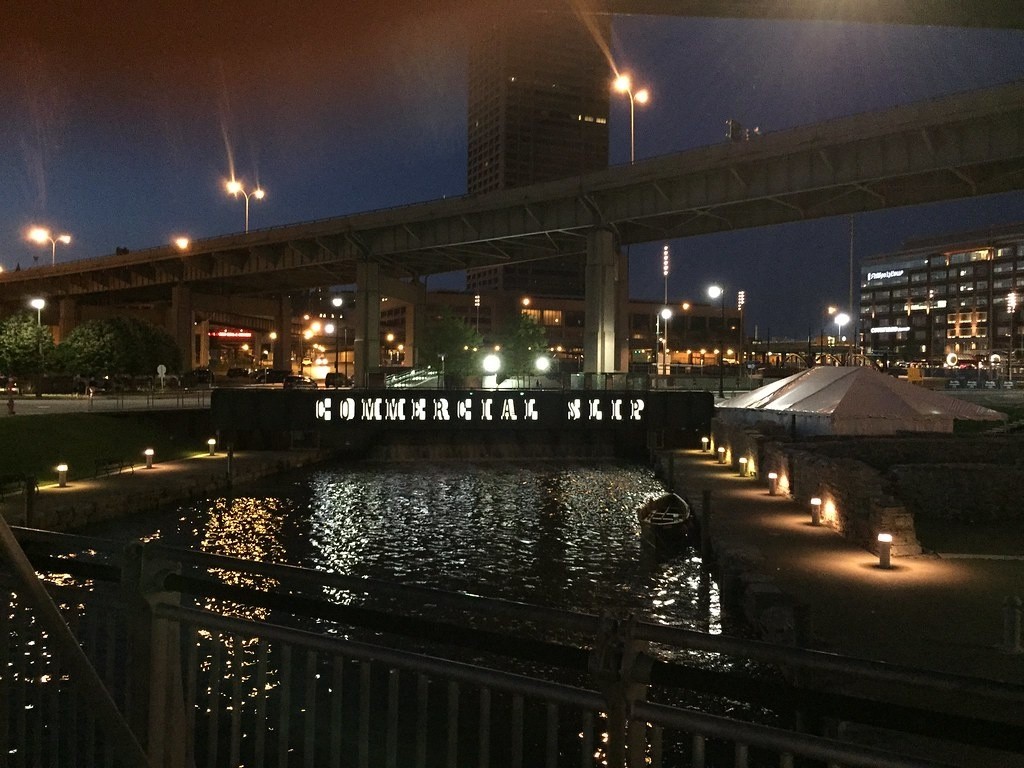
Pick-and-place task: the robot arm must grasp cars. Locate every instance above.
[0,376,20,395]
[228,368,248,377]
[73,367,214,395]
[325,373,354,387]
[251,368,293,383]
[283,374,318,389]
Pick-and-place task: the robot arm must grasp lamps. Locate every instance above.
[208,439,216,455]
[739,458,748,477]
[58,464,68,486]
[878,533,892,569]
[811,498,822,525]
[718,447,724,463]
[702,437,709,452]
[145,448,154,469]
[768,472,777,496]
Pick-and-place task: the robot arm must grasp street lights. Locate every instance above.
[709,285,726,398]
[735,292,745,330]
[333,296,343,392]
[610,72,648,168]
[1005,293,1018,382]
[298,314,309,373]
[226,180,264,235]
[681,302,689,354]
[28,225,71,270]
[474,295,479,333]
[654,310,671,386]
[31,299,45,330]
[835,315,848,343]
[664,245,670,307]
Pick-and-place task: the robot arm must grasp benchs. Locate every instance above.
[94,457,134,478]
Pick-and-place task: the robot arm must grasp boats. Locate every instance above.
[637,492,692,535]
